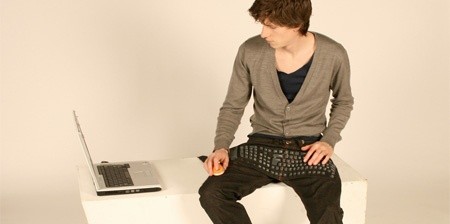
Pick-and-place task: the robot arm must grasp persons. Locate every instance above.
[196,1,355,224]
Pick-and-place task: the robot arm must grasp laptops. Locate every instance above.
[73,110,162,196]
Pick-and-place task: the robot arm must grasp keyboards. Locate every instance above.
[200,144,338,181]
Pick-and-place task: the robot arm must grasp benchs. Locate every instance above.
[74,150,367,224]
